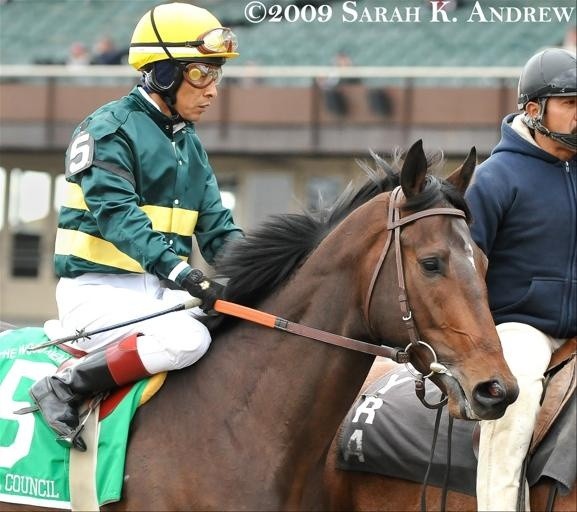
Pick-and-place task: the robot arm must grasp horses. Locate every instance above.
[326,336,577,511]
[0,138,519,512]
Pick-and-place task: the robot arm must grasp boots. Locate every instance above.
[29,329,156,450]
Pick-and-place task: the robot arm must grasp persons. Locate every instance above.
[87,36,123,65]
[555,25,576,52]
[317,50,359,119]
[29,3,247,452]
[462,44,575,511]
[67,41,91,66]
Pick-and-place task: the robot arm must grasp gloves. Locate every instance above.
[184,269,228,314]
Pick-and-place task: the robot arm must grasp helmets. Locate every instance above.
[517,48,577,106]
[127,3,239,72]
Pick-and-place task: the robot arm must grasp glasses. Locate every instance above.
[184,59,222,89]
[128,27,238,52]
